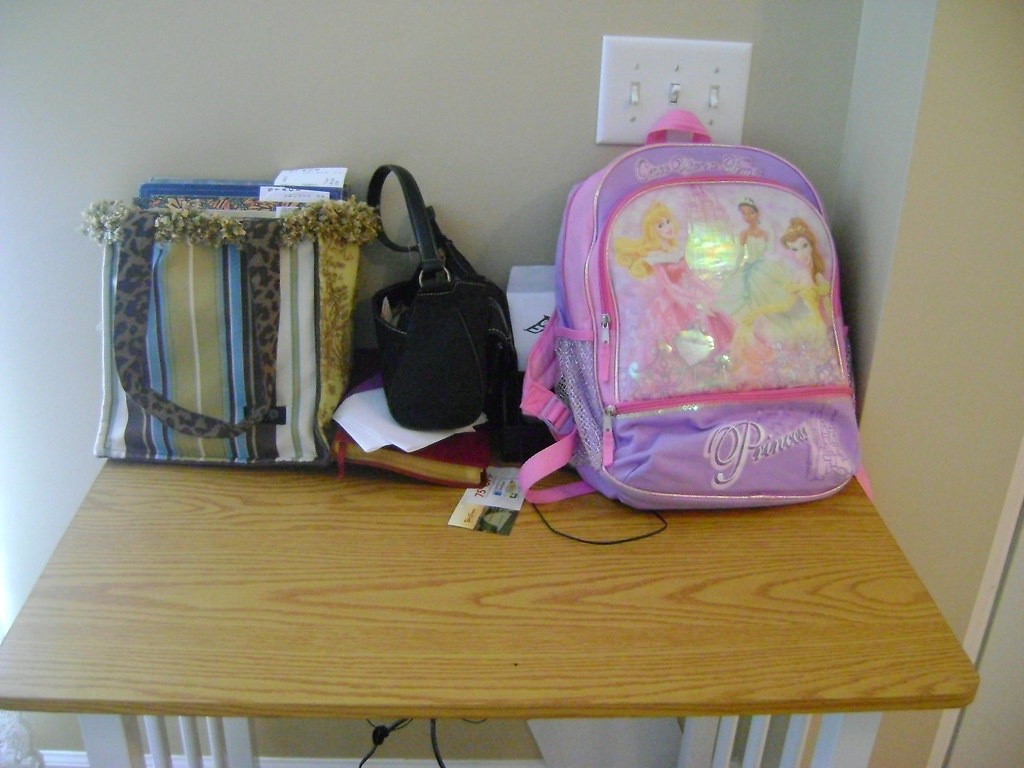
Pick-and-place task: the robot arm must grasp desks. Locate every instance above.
[0,361,981,768]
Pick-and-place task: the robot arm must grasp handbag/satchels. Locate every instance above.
[366,162,503,431]
[87,198,371,468]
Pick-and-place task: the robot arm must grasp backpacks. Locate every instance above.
[511,107,870,506]
